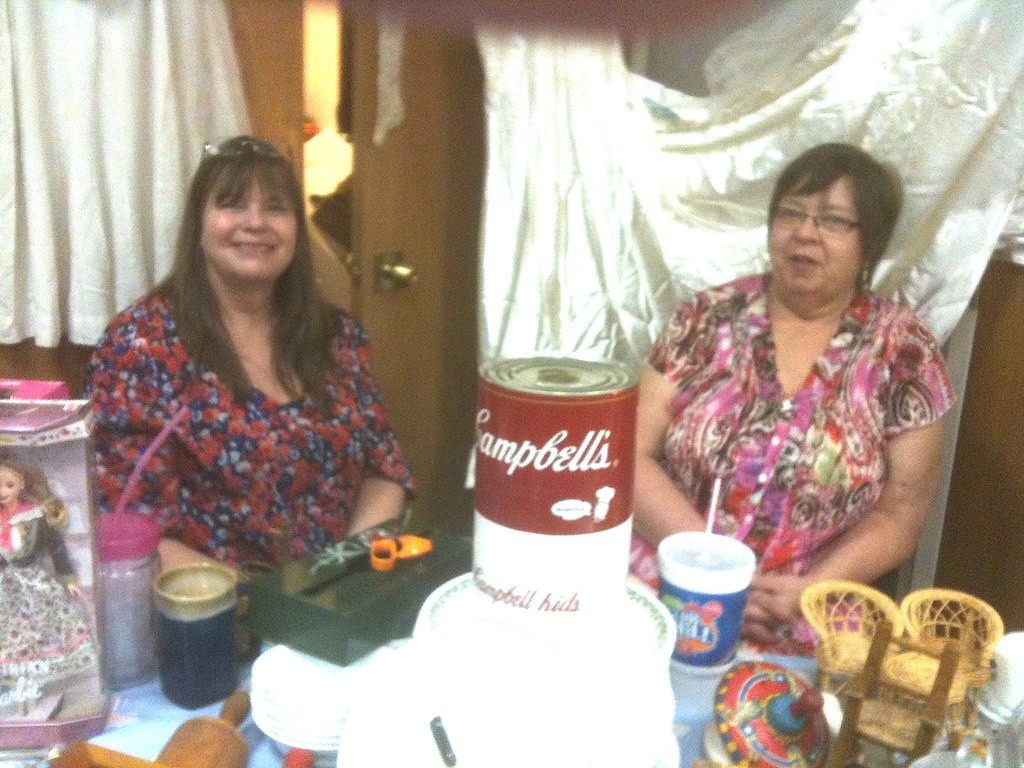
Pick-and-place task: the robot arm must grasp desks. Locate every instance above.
[0,651,823,768]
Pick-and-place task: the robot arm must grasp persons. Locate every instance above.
[0,450,95,715]
[81,135,416,577]
[628,142,957,659]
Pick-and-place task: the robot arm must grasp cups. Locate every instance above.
[656,530,756,675]
[153,561,240,711]
[97,511,163,689]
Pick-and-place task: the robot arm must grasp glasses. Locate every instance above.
[201,136,289,169]
[771,204,865,235]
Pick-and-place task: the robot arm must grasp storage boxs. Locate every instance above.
[242,514,474,670]
[0,379,113,749]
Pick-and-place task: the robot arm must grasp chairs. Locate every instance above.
[800,579,1006,768]
